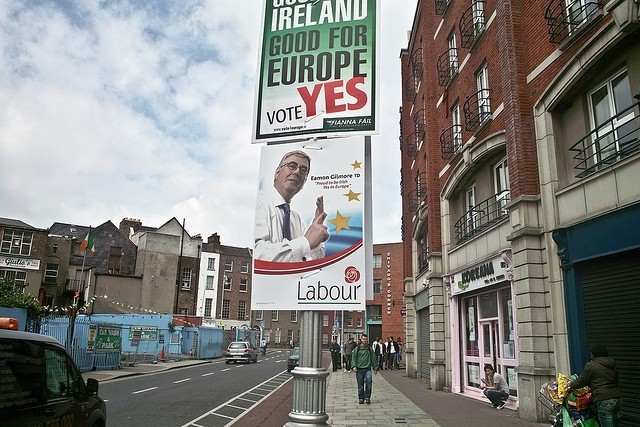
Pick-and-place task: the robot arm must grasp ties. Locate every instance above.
[280,203,291,240]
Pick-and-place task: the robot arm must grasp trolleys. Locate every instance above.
[537,378,597,427]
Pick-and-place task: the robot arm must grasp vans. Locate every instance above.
[0,329,106,426]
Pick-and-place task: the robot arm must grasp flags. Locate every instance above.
[80,224,95,254]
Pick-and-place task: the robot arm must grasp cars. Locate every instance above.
[225,342,257,364]
[287,347,299,372]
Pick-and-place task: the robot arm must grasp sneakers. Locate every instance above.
[359,399,364,404]
[497,400,506,410]
[366,398,370,404]
[490,403,496,408]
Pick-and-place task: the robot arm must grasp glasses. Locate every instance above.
[281,162,308,173]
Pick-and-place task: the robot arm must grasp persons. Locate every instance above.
[254,149,330,263]
[571,344,624,426]
[396,336,402,365]
[358,338,361,343]
[387,335,399,370]
[290,338,293,348]
[351,333,378,403]
[350,336,357,348]
[479,363,509,409]
[329,339,341,372]
[399,340,405,366]
[383,336,390,369]
[259,338,267,352]
[375,337,385,370]
[372,336,379,369]
[238,337,243,340]
[343,337,354,372]
